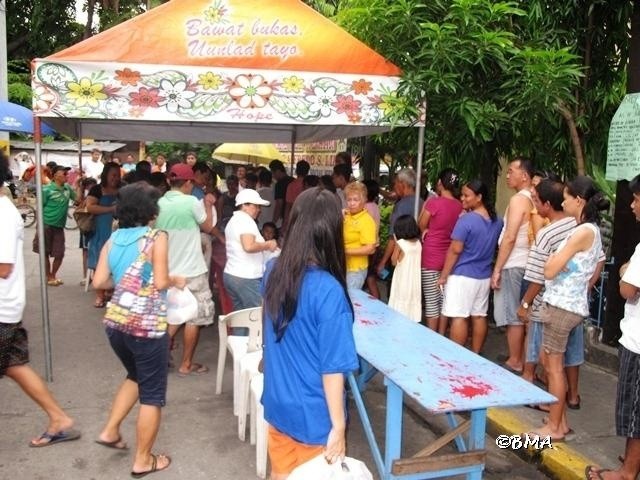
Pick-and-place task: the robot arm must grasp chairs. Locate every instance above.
[215,307,262,416]
[238,350,262,441]
[250,376,267,479]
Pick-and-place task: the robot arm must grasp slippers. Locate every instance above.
[585,464,615,480]
[519,417,573,444]
[132,453,170,479]
[566,390,582,410]
[94,434,131,451]
[496,352,548,388]
[30,428,82,447]
[525,405,550,413]
[176,363,210,378]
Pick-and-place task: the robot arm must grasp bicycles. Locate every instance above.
[14,198,81,231]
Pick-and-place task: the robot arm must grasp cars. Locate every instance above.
[350,158,413,183]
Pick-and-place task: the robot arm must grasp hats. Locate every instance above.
[234,188,271,207]
[167,162,197,180]
[51,165,71,176]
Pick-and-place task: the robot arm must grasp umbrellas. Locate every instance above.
[212,141,289,165]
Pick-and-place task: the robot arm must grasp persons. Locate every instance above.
[261,186,361,480]
[585,173,639,480]
[29,148,612,444]
[0,151,82,448]
[89,180,189,478]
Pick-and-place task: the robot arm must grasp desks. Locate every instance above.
[212,239,233,335]
[344,288,558,480]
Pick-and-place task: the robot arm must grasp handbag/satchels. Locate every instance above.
[72,200,97,238]
[102,227,169,339]
